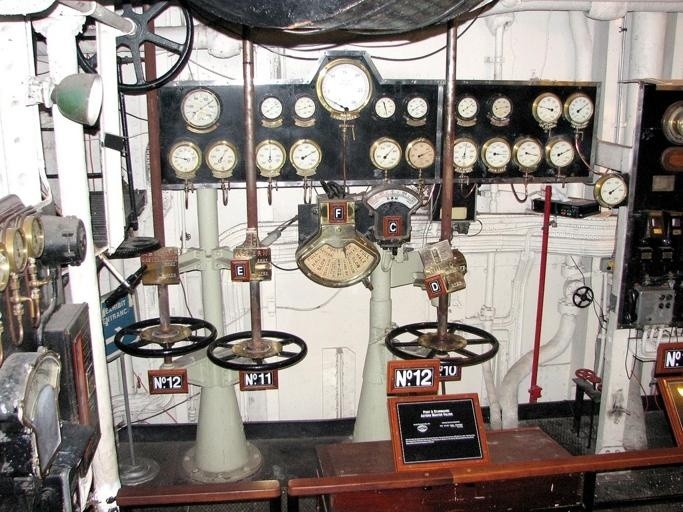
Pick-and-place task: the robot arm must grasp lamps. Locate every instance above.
[24,70,102,125]
